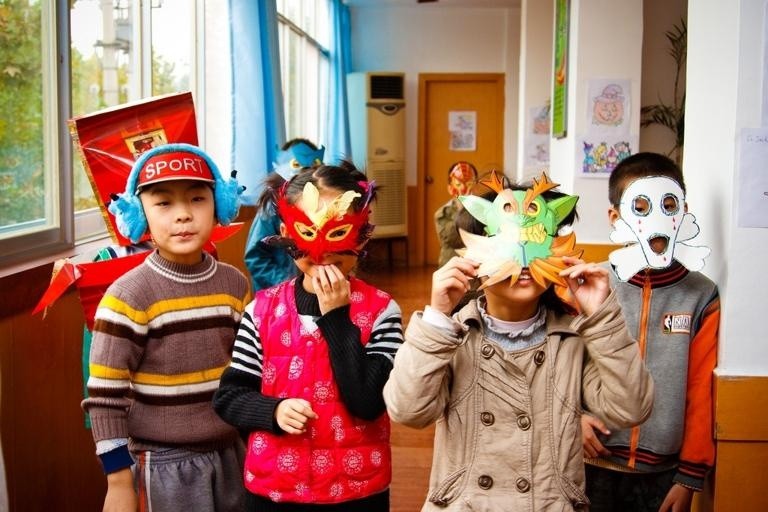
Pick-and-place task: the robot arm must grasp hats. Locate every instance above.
[133,151,215,195]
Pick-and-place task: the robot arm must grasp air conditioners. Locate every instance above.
[346,70,409,239]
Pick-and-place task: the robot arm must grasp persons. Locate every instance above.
[432,161,479,268]
[81,146,256,512]
[242,137,325,295]
[381,169,655,512]
[580,150,720,512]
[210,161,405,512]
